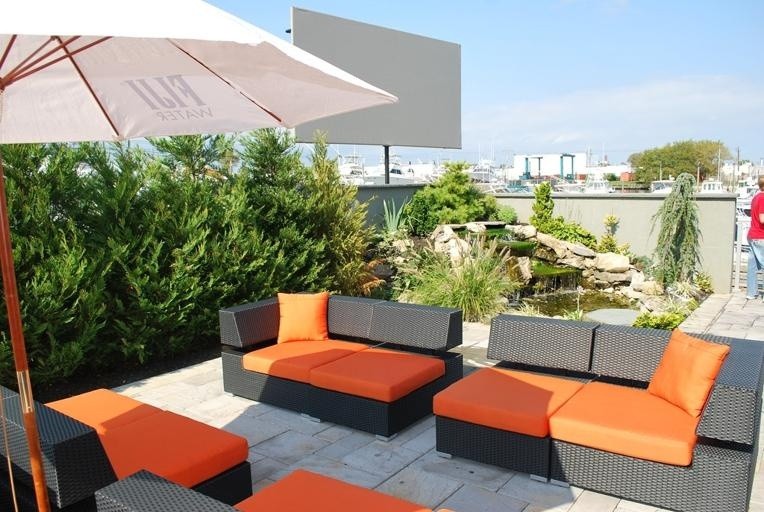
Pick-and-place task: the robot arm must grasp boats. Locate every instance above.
[330,143,725,195]
[725,176,758,266]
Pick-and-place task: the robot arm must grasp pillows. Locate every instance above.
[276,291,330,343]
[646,329,730,417]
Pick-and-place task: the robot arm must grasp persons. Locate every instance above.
[745,175,764,300]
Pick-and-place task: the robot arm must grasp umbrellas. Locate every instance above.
[0,0,399,510]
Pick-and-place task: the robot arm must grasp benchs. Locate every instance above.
[94,469,457,512]
[433,314,764,512]
[219,294,463,437]
[0,383,253,512]
